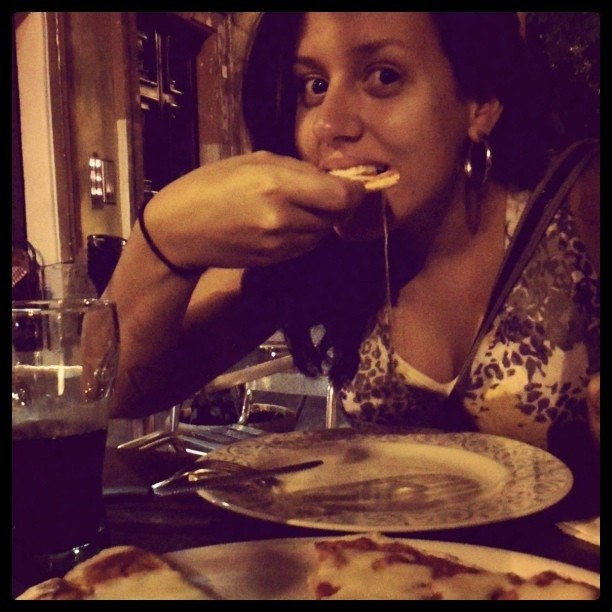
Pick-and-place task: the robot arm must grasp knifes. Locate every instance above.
[155,458,324,495]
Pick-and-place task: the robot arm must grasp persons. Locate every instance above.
[79,11,601,481]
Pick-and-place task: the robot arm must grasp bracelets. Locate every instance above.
[137,190,209,286]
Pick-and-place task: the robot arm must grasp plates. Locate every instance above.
[18,533,602,604]
[189,424,576,538]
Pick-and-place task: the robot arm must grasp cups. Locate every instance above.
[239,327,312,436]
[10,298,120,558]
[129,403,180,441]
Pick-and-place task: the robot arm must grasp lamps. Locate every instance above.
[88,151,105,209]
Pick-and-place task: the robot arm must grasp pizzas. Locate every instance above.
[328,169,400,200]
[311,537,598,600]
[16,543,212,600]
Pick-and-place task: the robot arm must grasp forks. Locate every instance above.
[103,460,283,495]
